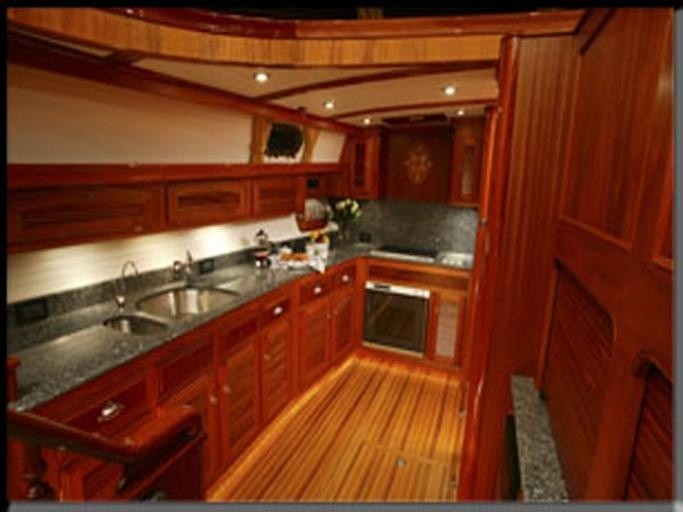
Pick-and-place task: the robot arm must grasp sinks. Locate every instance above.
[134,282,240,323]
[102,313,170,339]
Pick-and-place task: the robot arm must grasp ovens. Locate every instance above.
[362,280,430,359]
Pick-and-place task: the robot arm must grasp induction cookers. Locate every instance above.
[369,240,444,263]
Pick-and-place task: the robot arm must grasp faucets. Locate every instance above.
[111,259,140,311]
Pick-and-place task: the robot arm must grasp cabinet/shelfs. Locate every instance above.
[345,128,381,199]
[9,349,151,503]
[151,298,261,493]
[426,288,465,370]
[260,281,294,424]
[9,175,346,242]
[294,259,361,392]
[449,120,482,207]
